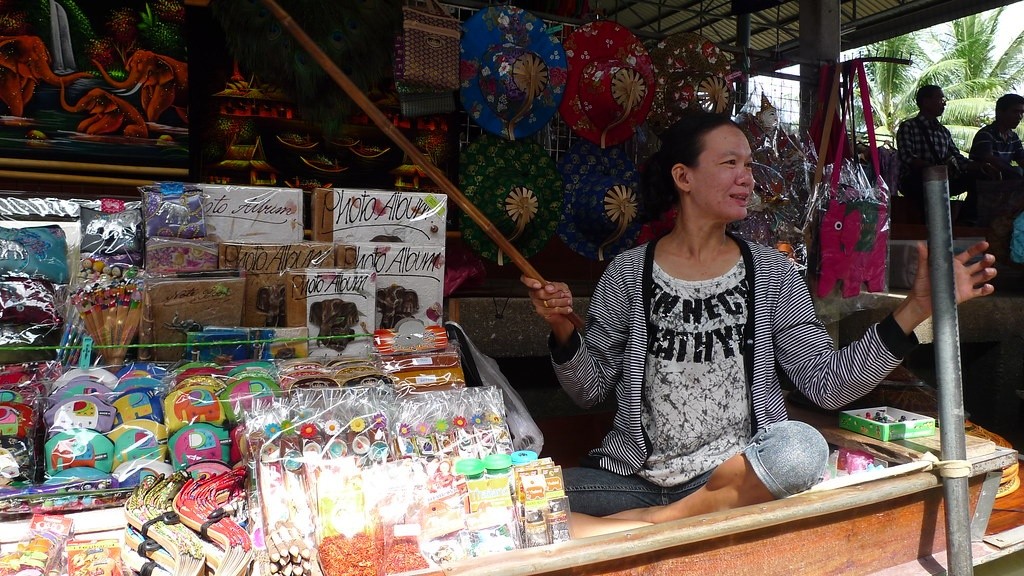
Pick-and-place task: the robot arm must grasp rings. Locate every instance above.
[544,301,548,307]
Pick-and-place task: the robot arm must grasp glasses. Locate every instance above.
[934,96,948,105]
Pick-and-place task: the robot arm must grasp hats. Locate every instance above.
[458,4,741,265]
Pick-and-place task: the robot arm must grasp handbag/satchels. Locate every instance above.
[442,320,544,459]
[0,360,395,499]
[808,55,889,300]
[395,0,460,117]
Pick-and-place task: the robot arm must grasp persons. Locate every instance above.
[968,94,1024,177]
[521,111,997,540]
[897,86,1002,199]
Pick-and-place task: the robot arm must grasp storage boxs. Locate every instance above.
[839,406,935,442]
